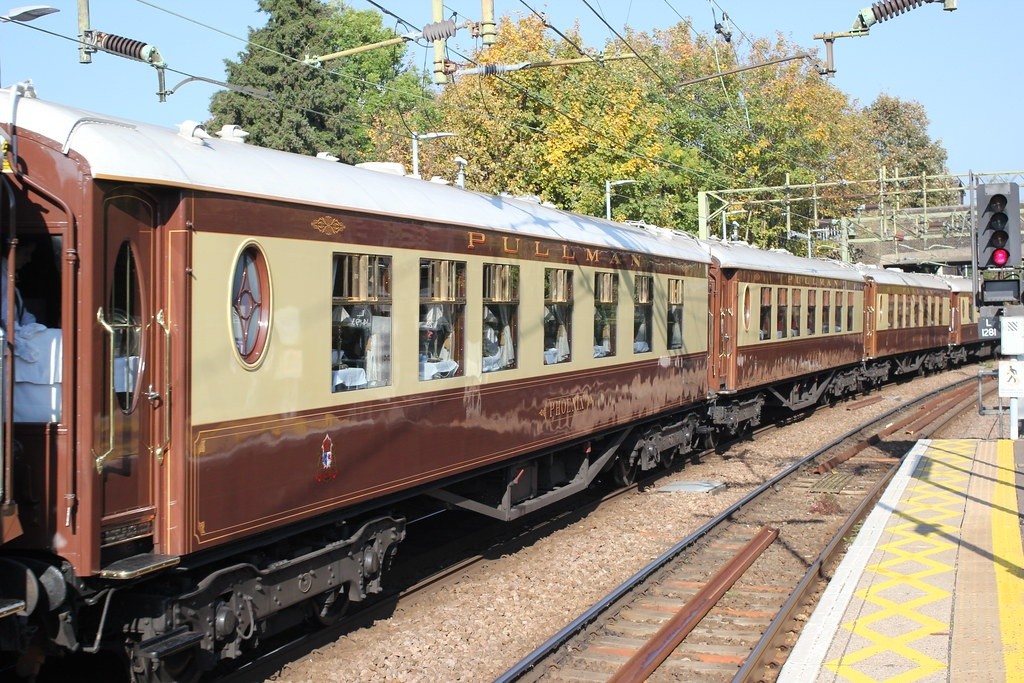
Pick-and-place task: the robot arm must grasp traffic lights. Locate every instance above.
[976,181,1024,270]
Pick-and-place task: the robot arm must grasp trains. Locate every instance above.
[0,81,1001,683]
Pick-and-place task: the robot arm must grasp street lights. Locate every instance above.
[411,131,456,176]
[604,179,639,220]
[721,209,749,240]
[805,227,829,259]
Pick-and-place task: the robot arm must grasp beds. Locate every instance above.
[482,313,502,371]
[595,307,610,357]
[420,321,460,381]
[332,304,373,392]
